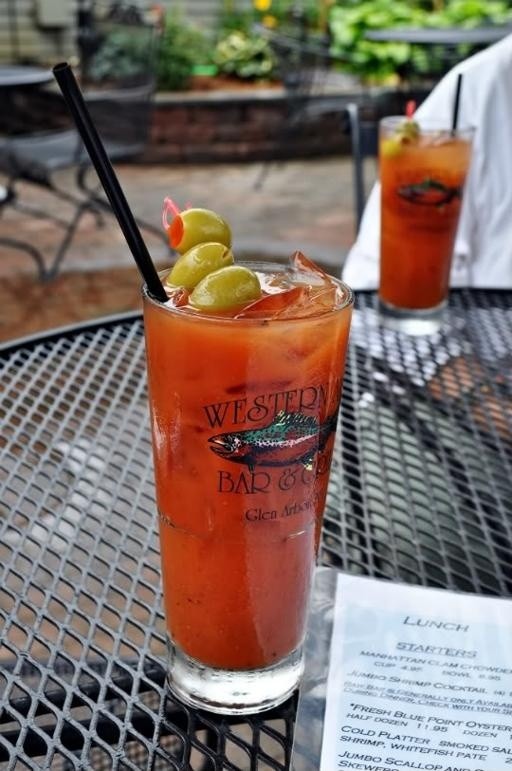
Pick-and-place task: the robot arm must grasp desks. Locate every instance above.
[2,62,60,212]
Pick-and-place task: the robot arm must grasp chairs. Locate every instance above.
[1,19,179,278]
[1,148,49,274]
[253,26,418,240]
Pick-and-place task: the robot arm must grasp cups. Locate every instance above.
[378,117,478,338]
[142,259,355,719]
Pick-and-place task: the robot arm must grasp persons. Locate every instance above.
[319,27,512,585]
